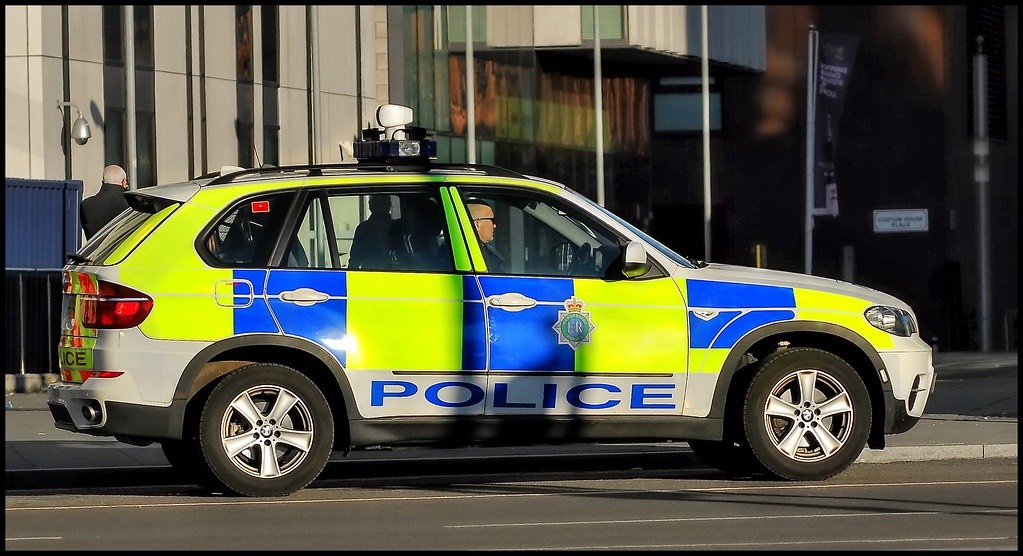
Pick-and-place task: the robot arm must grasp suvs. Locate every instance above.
[46,103,938,499]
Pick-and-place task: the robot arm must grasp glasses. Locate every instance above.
[125,179,130,188]
[474,218,496,224]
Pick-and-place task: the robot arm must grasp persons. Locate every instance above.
[348,194,398,270]
[80,165,131,241]
[463,199,497,273]
